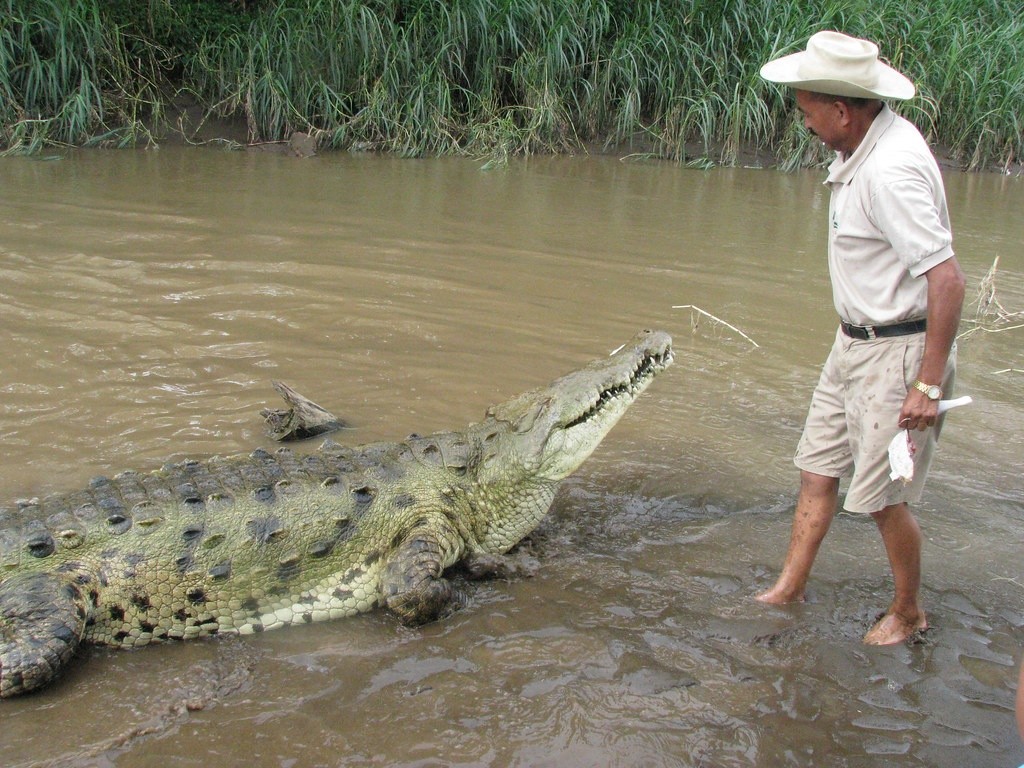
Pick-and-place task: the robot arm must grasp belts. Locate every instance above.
[838,318,927,340]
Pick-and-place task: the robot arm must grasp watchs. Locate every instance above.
[913,380,943,400]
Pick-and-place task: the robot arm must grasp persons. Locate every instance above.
[754,31,965,647]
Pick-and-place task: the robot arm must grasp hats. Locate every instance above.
[760,31,915,101]
